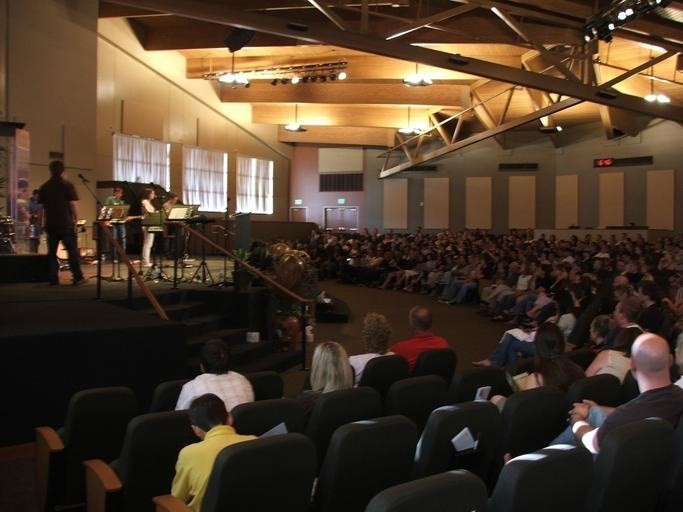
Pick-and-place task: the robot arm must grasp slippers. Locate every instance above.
[472,361,491,369]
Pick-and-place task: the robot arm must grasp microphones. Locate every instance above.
[150,182,157,190]
[77,173,89,182]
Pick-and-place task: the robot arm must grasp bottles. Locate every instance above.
[28,224,35,238]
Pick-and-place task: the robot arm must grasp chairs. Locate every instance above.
[473,262,497,296]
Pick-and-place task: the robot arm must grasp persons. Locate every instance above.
[253,221,682,366]
[104,185,135,261]
[157,193,178,261]
[7,179,31,235]
[170,308,683,512]
[27,190,42,253]
[37,159,87,285]
[140,187,158,268]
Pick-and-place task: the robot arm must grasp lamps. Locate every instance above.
[201,28,433,136]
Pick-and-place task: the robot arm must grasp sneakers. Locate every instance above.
[74,278,88,285]
[142,263,153,267]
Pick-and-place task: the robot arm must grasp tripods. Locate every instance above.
[188,224,214,284]
[90,206,188,283]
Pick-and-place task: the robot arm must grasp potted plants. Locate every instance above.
[229,248,263,290]
